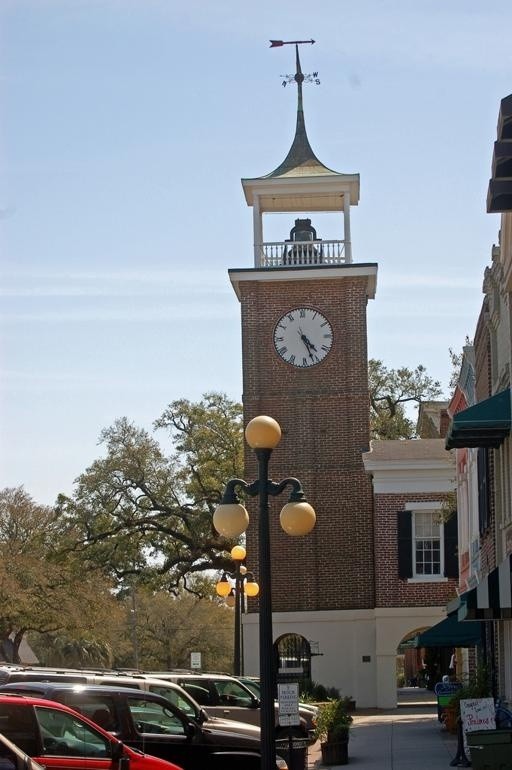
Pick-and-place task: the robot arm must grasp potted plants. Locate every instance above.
[311,695,354,765]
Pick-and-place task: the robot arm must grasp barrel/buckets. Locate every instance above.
[435,681,463,725]
[463,730,512,770]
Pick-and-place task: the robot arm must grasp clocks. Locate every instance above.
[272,308,334,368]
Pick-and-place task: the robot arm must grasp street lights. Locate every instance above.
[239,565,248,615]
[209,407,318,770]
[213,542,259,677]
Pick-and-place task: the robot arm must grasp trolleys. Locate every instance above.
[434,679,463,732]
